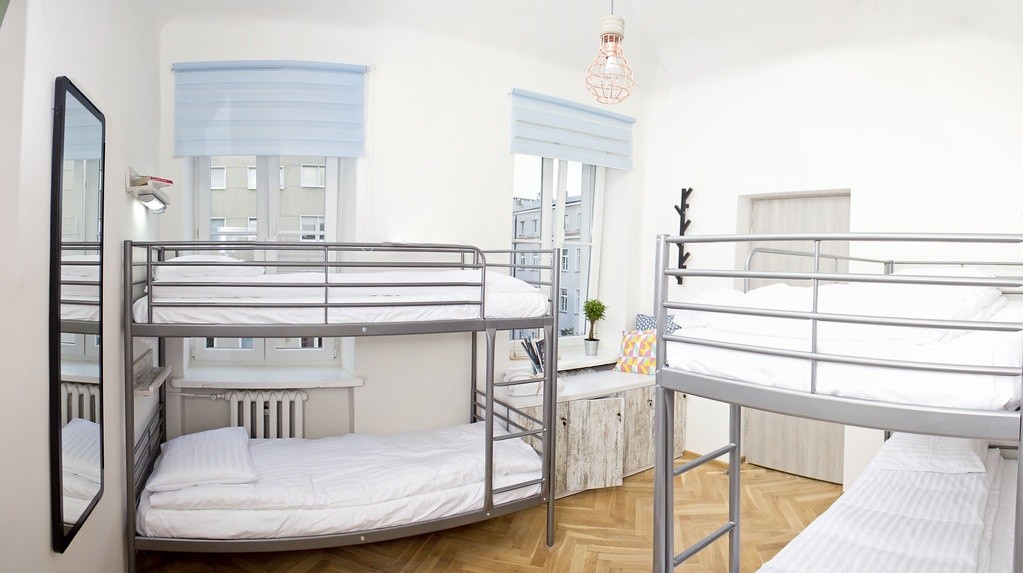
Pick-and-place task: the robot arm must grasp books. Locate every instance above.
[521,335,545,374]
[130,173,174,189]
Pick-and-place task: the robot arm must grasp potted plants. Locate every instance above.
[582,299,606,358]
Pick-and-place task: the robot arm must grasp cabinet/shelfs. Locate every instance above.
[517,383,685,501]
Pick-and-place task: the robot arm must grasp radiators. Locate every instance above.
[223,390,309,439]
[61,380,100,428]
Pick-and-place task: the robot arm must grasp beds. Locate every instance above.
[658,235,1023,572]
[123,242,561,573]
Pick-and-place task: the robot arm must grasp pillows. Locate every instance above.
[874,430,988,472]
[61,417,100,480]
[636,313,682,336]
[612,326,658,372]
[153,253,240,278]
[145,425,247,492]
[886,269,1003,313]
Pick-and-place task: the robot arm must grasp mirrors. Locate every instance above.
[50,75,107,553]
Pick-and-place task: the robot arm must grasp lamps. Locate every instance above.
[138,195,167,214]
[586,0,634,104]
